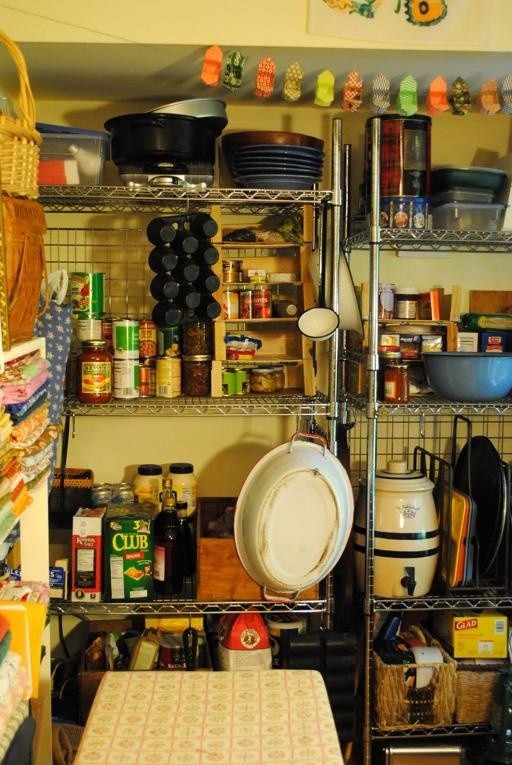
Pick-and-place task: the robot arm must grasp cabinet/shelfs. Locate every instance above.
[343,113,512,765]
[0,192,53,763]
[36,116,345,763]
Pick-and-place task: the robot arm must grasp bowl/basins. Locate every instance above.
[420,351,512,405]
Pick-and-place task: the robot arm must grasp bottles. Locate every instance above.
[273,282,297,315]
[110,481,130,490]
[91,491,112,506]
[252,289,273,318]
[173,501,197,577]
[359,281,396,321]
[111,317,139,359]
[78,338,112,403]
[223,292,240,318]
[74,318,100,340]
[92,483,110,491]
[164,462,198,519]
[114,359,140,399]
[222,367,235,396]
[155,499,183,594]
[232,368,250,395]
[134,463,162,502]
[240,290,252,319]
[222,259,244,283]
[140,359,155,400]
[111,487,134,504]
[141,317,157,357]
[70,272,104,318]
[395,286,421,321]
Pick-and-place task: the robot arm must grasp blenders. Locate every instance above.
[365,112,434,198]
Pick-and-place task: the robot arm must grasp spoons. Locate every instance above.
[297,199,342,343]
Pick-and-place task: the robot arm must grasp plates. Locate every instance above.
[229,144,326,194]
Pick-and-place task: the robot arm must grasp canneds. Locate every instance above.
[183,318,212,354]
[160,325,182,357]
[384,364,408,405]
[183,358,209,396]
[378,350,403,401]
[155,359,182,398]
[250,368,276,392]
[269,366,288,392]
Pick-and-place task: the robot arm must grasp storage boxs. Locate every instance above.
[196,496,323,602]
[370,640,455,732]
[453,658,506,729]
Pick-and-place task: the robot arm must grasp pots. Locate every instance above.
[235,431,355,606]
[104,111,222,175]
